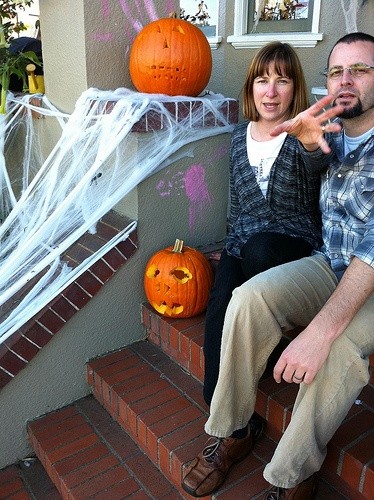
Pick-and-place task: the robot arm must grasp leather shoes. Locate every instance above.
[182,423,252,497]
[262,473,319,500]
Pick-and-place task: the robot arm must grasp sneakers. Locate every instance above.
[248,411,267,440]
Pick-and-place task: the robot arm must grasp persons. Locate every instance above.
[182,32,374,500]
[204,41,344,410]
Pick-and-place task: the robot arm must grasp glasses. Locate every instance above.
[320,62,374,80]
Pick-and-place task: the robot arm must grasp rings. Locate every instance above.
[294,376,302,381]
[322,124,326,133]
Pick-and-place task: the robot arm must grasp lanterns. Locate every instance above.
[143,239,212,319]
[129,12,212,97]
